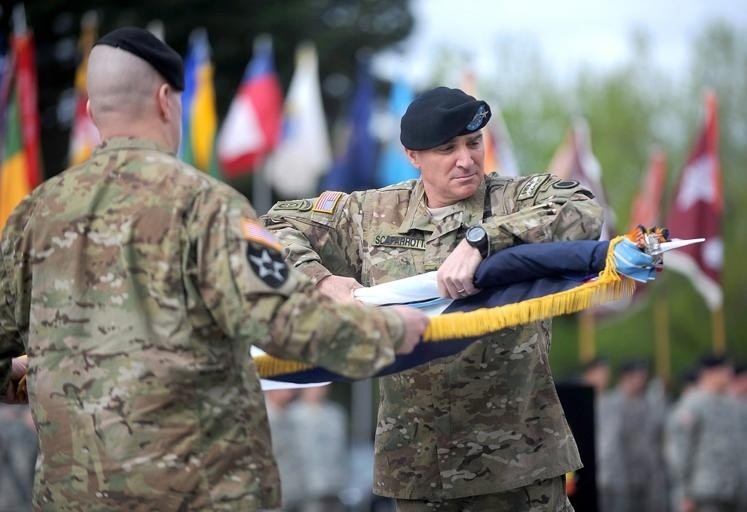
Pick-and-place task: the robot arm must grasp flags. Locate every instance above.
[459,72,512,179]
[0,31,39,239]
[625,136,664,234]
[649,89,729,312]
[253,225,677,404]
[540,116,648,319]
[172,27,421,216]
[62,19,105,167]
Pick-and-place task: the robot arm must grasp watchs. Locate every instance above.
[465,225,488,258]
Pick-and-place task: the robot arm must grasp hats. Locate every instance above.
[582,349,746,379]
[92,26,185,92]
[400,86,492,151]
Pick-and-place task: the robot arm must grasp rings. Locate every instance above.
[456,289,464,294]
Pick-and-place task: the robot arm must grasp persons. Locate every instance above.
[0,345,37,511]
[259,86,615,511]
[566,347,746,511]
[0,25,429,511]
[259,378,348,511]
